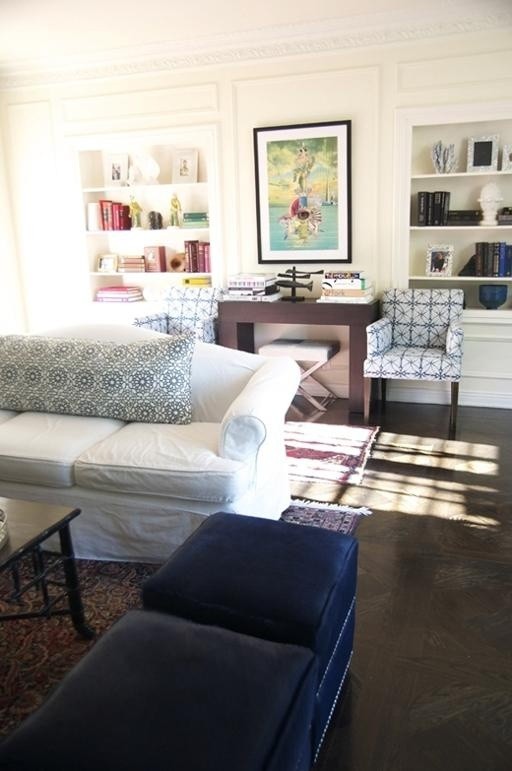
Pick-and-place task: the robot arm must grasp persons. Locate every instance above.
[111,164,121,180]
[179,158,189,176]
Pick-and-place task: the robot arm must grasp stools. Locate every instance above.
[140,510,358,760]
[258,339,341,412]
[1,607,317,770]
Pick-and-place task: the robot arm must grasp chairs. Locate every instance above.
[362,290,465,430]
[132,287,222,344]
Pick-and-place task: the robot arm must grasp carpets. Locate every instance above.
[1,497,373,737]
[286,420,380,486]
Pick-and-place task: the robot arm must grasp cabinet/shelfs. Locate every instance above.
[394,102,512,310]
[65,125,227,304]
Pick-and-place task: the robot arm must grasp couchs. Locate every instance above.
[0,318,301,565]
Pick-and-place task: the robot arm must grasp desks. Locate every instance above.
[217,299,380,413]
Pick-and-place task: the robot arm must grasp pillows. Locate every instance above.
[0,332,192,426]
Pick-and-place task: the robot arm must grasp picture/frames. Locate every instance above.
[254,121,353,263]
[467,135,499,172]
[172,149,198,184]
[425,244,453,277]
[103,154,129,188]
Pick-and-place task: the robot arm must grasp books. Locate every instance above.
[418,190,511,277]
[316,271,376,304]
[88,200,211,302]
[223,272,282,303]
